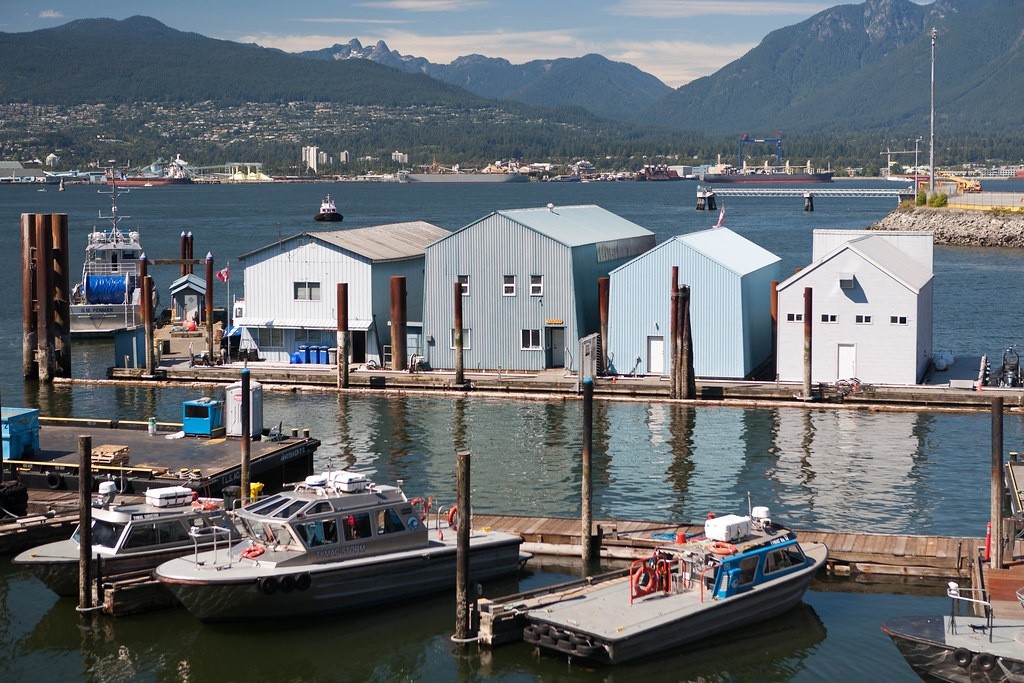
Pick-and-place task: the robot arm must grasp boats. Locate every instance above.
[523,492,828,663]
[69,159,159,337]
[12,462,270,598]
[881,582,1024,683]
[59,176,65,192]
[314,194,343,222]
[143,183,153,187]
[151,450,534,622]
[704,131,836,183]
[105,176,198,186]
[409,159,528,183]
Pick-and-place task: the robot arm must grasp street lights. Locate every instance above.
[915,139,922,202]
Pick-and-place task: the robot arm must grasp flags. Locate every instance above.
[216,266,230,285]
[712,205,727,228]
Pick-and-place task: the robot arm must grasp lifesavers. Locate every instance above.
[634,566,656,596]
[46,473,62,490]
[241,546,264,558]
[0,481,29,519]
[115,477,128,494]
[448,505,458,532]
[706,541,737,556]
[191,499,217,510]
[410,497,427,520]
